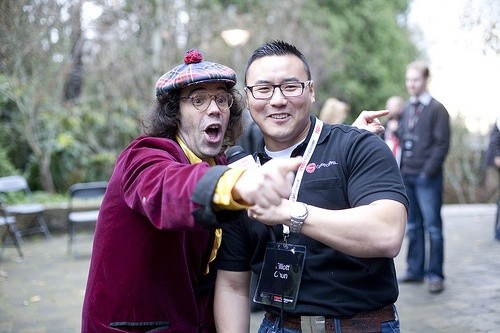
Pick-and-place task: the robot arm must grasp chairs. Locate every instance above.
[65,181,110,248]
[0,175,52,259]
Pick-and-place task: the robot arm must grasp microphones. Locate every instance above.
[224,145,276,244]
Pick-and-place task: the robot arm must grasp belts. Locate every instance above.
[265,306,395,333]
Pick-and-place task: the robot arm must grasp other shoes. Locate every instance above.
[428,272,442,292]
[398,272,417,282]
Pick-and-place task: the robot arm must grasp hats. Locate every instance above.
[155,48,237,95]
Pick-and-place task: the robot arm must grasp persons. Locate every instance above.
[212,39,413,333]
[82,49,389,333]
[319,60,500,294]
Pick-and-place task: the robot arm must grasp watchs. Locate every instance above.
[289,201,308,233]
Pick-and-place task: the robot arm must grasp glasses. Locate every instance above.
[176,92,234,111]
[247,81,310,99]
